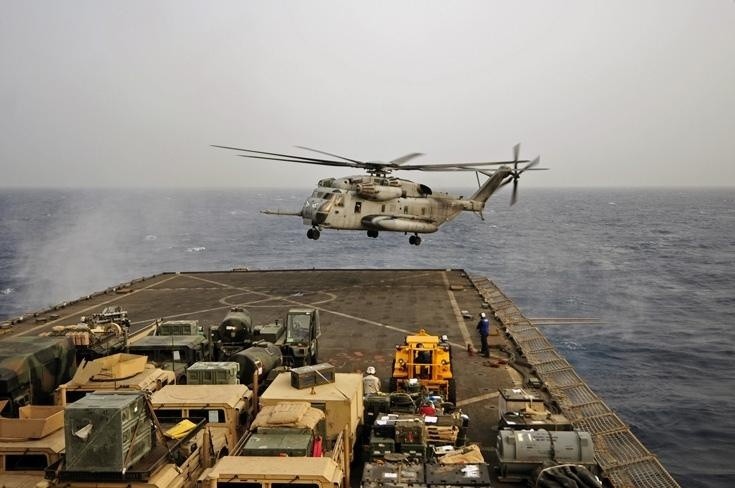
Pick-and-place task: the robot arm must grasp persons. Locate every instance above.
[363,365,382,395]
[475,312,491,358]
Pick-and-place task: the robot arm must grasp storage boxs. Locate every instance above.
[290,362,335,390]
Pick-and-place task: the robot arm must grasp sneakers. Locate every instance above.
[477,351,490,359]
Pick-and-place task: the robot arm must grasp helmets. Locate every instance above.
[479,312,486,319]
[365,365,377,375]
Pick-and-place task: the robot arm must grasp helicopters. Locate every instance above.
[209,142,550,246]
